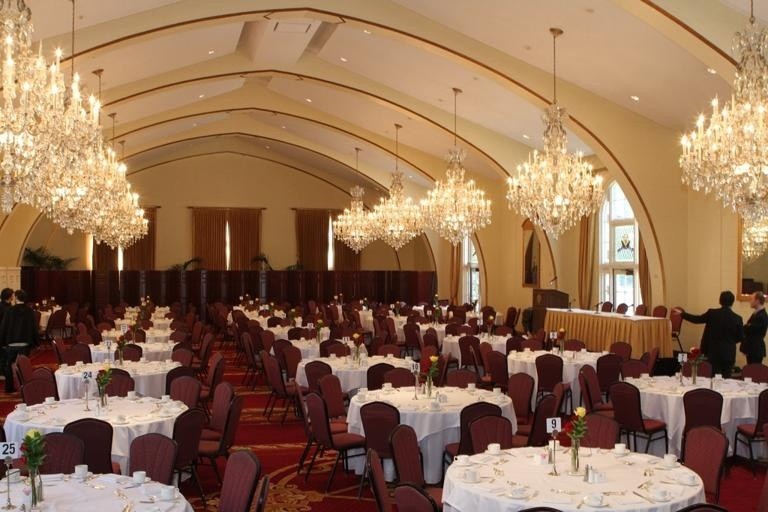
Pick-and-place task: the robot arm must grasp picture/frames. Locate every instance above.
[736,203,768,303]
[520,215,541,289]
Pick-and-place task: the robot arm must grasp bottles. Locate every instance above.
[583,464,594,483]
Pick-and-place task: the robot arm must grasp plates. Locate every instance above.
[452,461,472,466]
[652,495,672,502]
[461,478,481,483]
[485,450,504,455]
[584,496,610,508]
[679,480,700,486]
[662,462,681,468]
[506,490,534,499]
[612,448,630,456]
[545,446,563,451]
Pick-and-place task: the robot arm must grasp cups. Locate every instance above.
[549,440,559,449]
[512,486,528,496]
[511,348,609,362]
[664,454,677,466]
[15,391,183,424]
[357,383,508,411]
[589,492,603,505]
[299,336,349,347]
[0,465,179,512]
[462,469,480,481]
[625,373,767,395]
[328,352,412,369]
[682,472,696,484]
[615,444,626,453]
[59,329,178,377]
[488,444,500,453]
[447,332,511,343]
[655,489,669,500]
[454,455,469,464]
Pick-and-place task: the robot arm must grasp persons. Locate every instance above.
[739,291,767,365]
[0,289,39,394]
[671,291,743,380]
[0,287,15,381]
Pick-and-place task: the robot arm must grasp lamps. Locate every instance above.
[0,0,149,251]
[676,0,768,224]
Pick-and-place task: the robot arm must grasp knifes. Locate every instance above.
[634,491,656,504]
[504,451,515,457]
[577,497,588,509]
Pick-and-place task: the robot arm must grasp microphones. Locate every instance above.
[548,277,557,282]
